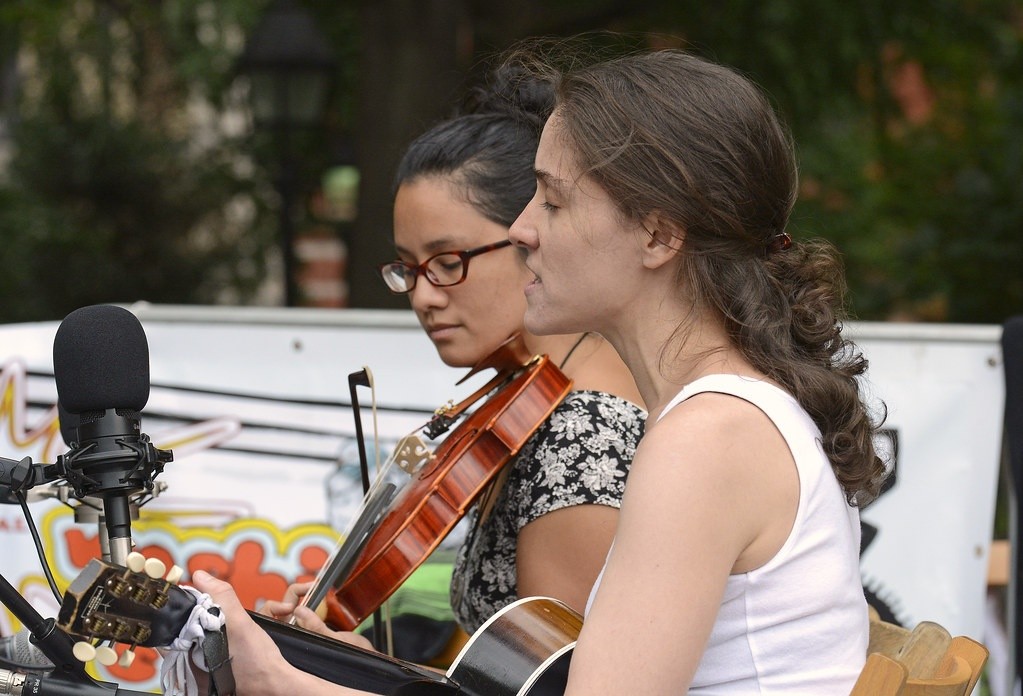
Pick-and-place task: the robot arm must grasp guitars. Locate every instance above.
[57,557,587,696]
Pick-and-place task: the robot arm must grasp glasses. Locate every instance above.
[378,239,513,293]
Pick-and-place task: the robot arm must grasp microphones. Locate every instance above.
[53,305,150,497]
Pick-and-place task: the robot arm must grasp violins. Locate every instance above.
[282,330,573,632]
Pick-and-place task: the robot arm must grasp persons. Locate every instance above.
[190,31,890,696]
[257,111,650,654]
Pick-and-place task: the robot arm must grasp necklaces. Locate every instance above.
[450,332,590,612]
